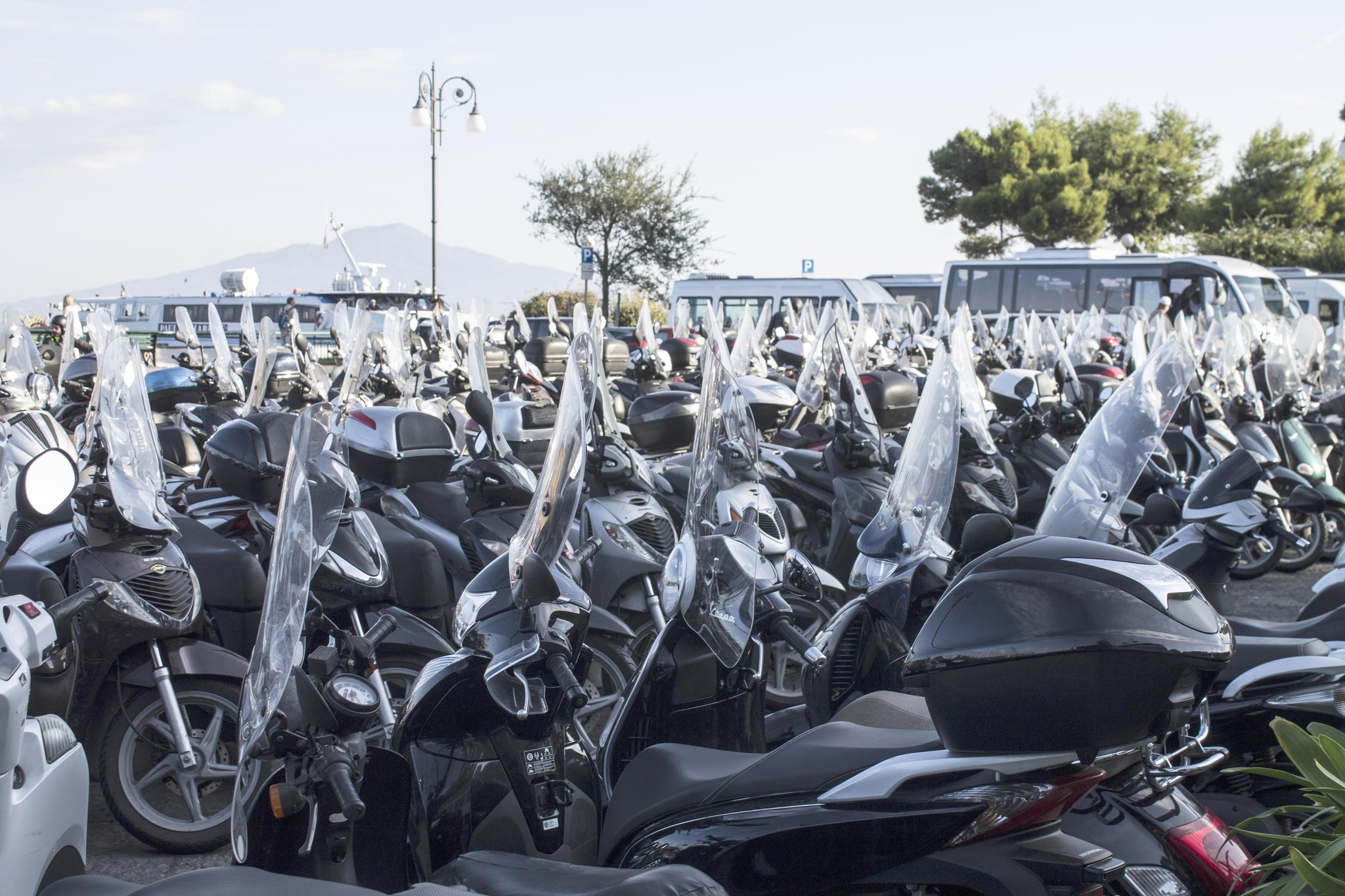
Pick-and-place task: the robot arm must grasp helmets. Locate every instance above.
[52,315,68,335]
[790,297,803,314]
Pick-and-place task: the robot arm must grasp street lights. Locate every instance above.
[410,60,487,300]
[1121,233,1136,254]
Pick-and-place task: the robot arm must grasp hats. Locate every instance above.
[1158,296,1174,307]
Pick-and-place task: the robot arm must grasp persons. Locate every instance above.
[1150,295,1174,331]
[368,299,383,312]
[429,299,472,339]
[43,316,93,354]
[766,297,802,340]
[1188,289,1214,322]
[278,297,297,346]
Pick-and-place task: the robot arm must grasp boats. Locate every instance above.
[49,210,473,355]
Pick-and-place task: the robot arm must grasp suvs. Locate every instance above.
[482,315,617,350]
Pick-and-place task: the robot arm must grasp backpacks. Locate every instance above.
[278,305,295,329]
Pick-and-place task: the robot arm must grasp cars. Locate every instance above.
[604,327,660,352]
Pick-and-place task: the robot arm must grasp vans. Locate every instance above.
[667,272,948,349]
[937,245,1344,341]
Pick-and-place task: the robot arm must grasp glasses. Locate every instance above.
[291,301,295,303]
[52,326,62,329]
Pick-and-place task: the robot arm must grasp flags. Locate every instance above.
[120,284,125,297]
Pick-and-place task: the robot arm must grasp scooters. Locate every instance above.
[0,303,1345,896]
